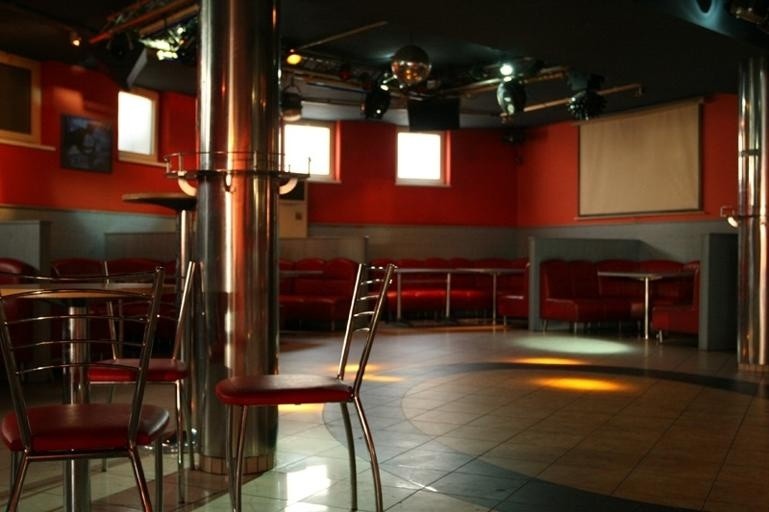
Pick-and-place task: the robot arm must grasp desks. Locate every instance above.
[393,268,524,327]
[278,270,335,280]
[121,187,201,453]
[597,271,694,341]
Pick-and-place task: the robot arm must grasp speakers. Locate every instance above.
[406,97,463,131]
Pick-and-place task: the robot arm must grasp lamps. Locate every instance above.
[287,45,302,66]
[283,84,302,122]
[112,45,148,91]
[568,88,604,122]
[362,86,391,121]
[497,79,527,116]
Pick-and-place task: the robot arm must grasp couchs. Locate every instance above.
[539,259,700,344]
[370,256,531,327]
[278,258,360,331]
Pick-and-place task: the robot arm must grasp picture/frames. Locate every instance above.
[60,113,114,174]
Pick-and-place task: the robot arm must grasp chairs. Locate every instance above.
[218,262,396,512]
[94,256,195,512]
